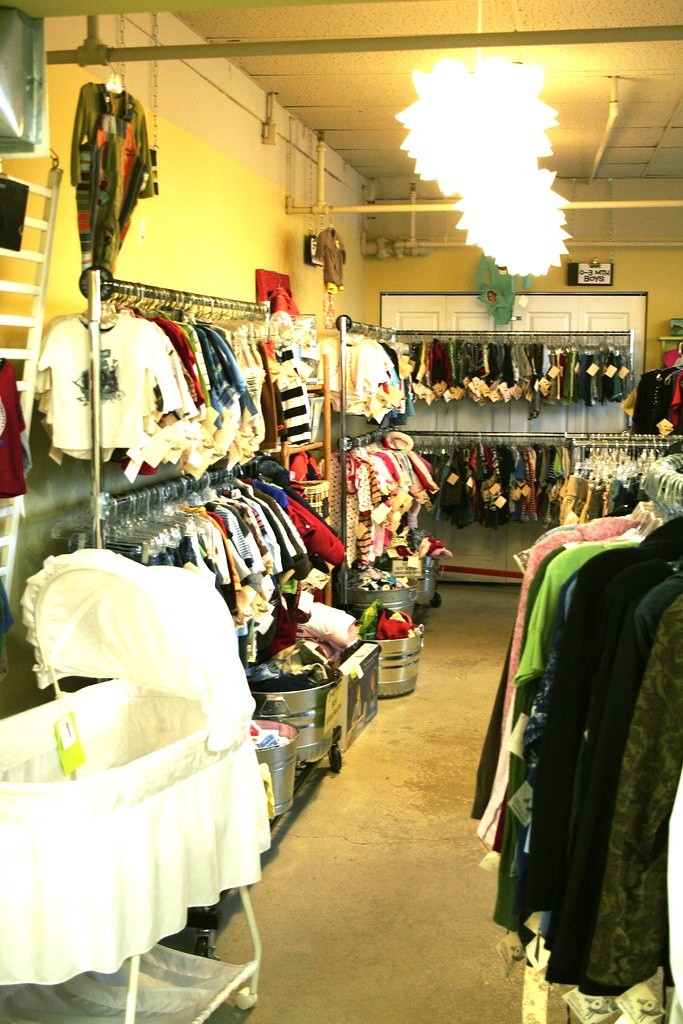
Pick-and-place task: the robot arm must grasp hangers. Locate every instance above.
[326,215,335,232]
[50,280,683,557]
[105,62,122,93]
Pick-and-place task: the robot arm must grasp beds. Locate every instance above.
[1,547,273,1024]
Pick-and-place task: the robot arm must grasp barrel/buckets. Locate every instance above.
[249,667,342,763]
[252,719,300,816]
[359,619,422,697]
[349,577,420,622]
[393,557,440,604]
